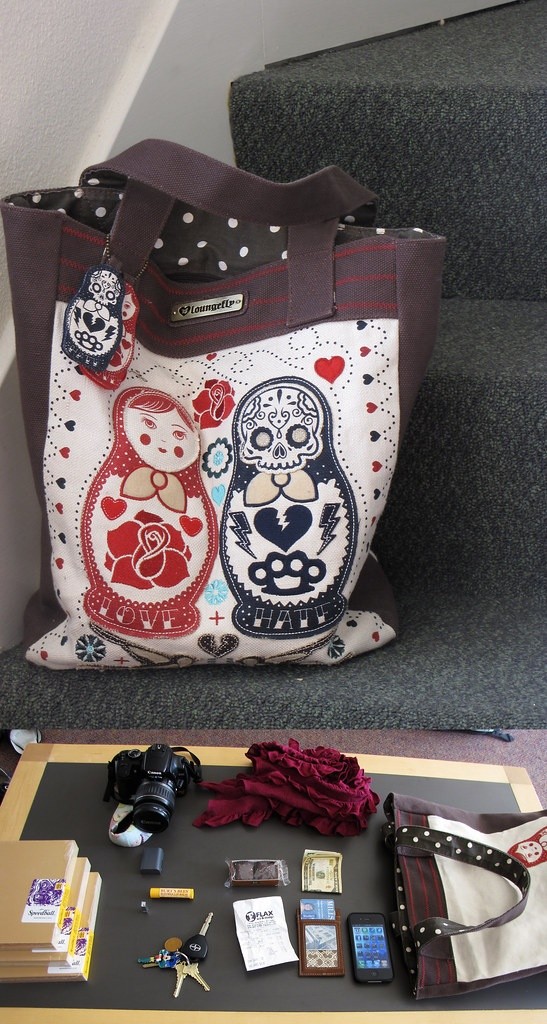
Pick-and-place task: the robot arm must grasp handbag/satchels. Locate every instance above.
[375,787,547,1003]
[3,136,449,678]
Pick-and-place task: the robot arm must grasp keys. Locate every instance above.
[140,911,217,999]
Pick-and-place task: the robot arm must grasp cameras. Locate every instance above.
[107,744,195,833]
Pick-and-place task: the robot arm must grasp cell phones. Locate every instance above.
[347,912,395,985]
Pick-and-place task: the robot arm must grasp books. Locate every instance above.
[1,840,103,986]
